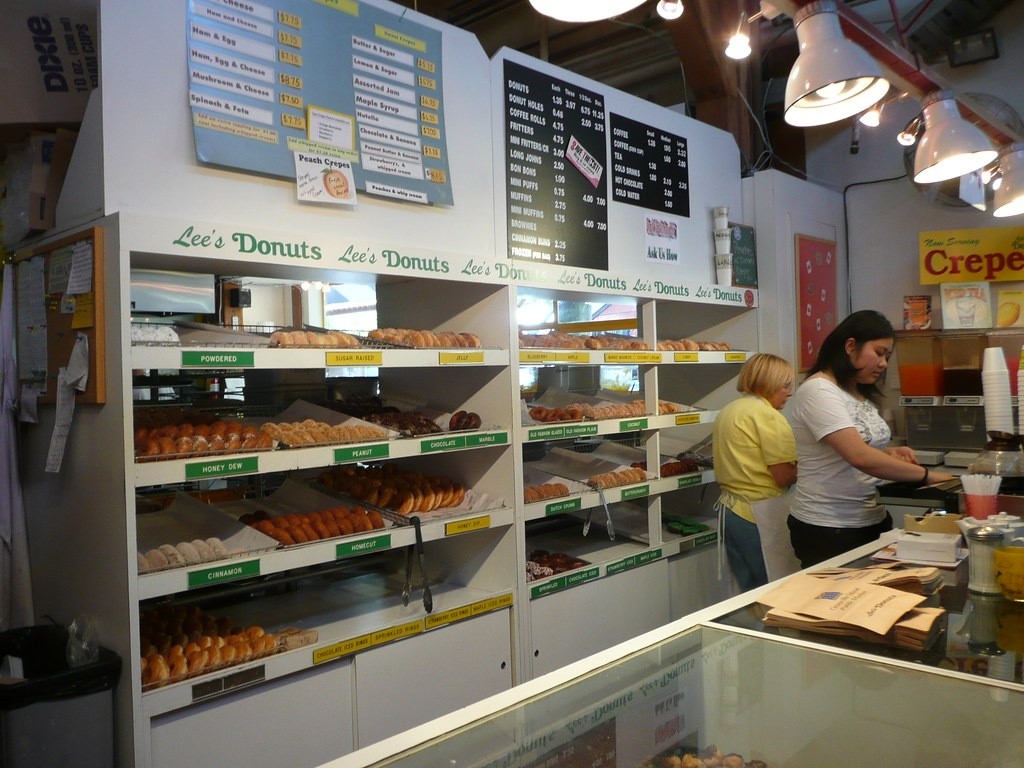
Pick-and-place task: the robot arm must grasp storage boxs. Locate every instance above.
[893,328,1024,396]
[896,530,962,564]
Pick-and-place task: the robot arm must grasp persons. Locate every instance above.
[712,352,798,595]
[787,309,958,570]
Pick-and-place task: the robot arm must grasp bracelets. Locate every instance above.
[920,467,928,482]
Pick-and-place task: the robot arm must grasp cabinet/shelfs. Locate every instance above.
[8,210,758,768]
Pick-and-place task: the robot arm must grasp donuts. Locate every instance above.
[129,323,483,687]
[515,329,730,580]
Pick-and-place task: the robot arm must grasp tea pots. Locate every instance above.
[955,511,1024,546]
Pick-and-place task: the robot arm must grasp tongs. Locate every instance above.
[583,483,615,541]
[699,464,713,504]
[402,516,433,614]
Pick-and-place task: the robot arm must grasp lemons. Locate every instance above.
[996,302,1019,328]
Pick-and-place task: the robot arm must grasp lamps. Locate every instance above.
[527,0,1024,218]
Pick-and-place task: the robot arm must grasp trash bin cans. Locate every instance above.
[0,624,122,768]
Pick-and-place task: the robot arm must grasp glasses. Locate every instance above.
[781,384,793,395]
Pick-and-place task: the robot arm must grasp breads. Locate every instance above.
[649,745,770,768]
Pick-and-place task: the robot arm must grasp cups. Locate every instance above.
[981,347,1014,443]
[1017,344,1024,436]
[995,598,1024,653]
[993,546,1024,602]
[963,494,998,521]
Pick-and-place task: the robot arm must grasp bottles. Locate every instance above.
[968,592,1006,656]
[966,527,1006,593]
